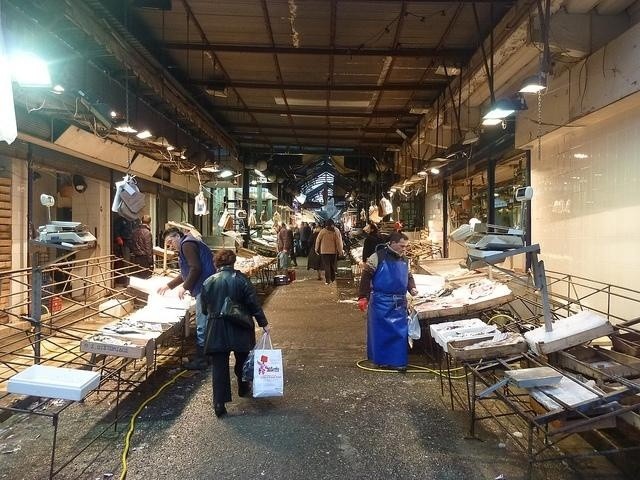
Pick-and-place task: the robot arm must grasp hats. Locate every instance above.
[162,227,179,238]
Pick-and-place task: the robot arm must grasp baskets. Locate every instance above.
[336,278,353,288]
[274,270,296,286]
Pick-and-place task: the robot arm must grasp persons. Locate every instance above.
[157,227,215,370]
[114,215,140,277]
[276,219,346,285]
[357,224,390,299]
[358,232,419,372]
[132,214,154,281]
[201,249,270,418]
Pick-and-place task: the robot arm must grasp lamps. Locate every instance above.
[164,145,234,178]
[392,168,439,193]
[109,108,152,142]
[480,72,547,127]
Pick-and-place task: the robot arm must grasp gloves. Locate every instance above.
[358,298,368,312]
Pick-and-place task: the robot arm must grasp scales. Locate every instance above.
[37,193,97,244]
[465,186,533,250]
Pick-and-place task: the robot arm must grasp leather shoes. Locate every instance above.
[215,406,226,417]
[183,358,209,369]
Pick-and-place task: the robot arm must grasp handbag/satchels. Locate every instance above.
[220,270,255,330]
[253,332,283,398]
